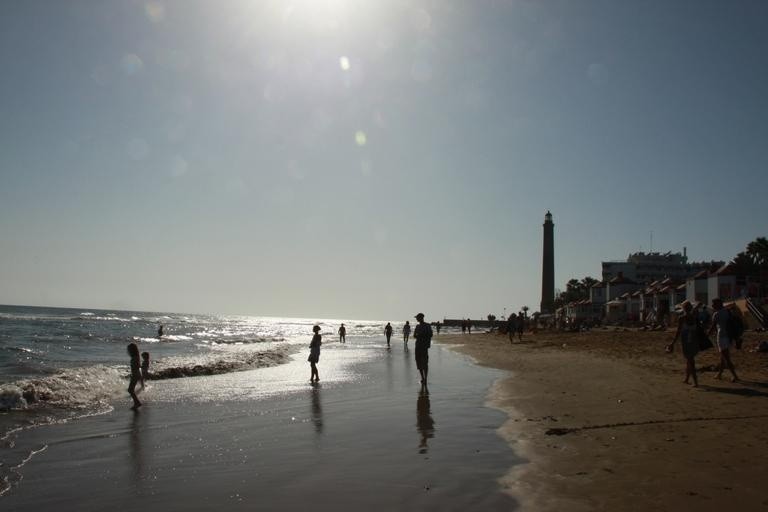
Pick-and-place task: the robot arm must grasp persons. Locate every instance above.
[157,325,163,338]
[402,321,411,344]
[462,320,466,334]
[308,325,321,383]
[436,321,440,332]
[413,313,433,383]
[415,384,436,452]
[339,324,345,342]
[708,300,743,382]
[467,319,471,335]
[664,299,711,388]
[141,351,149,390]
[127,344,142,408]
[384,323,392,346]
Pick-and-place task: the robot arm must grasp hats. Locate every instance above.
[414,313,424,318]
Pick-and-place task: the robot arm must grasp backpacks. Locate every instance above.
[725,308,745,339]
